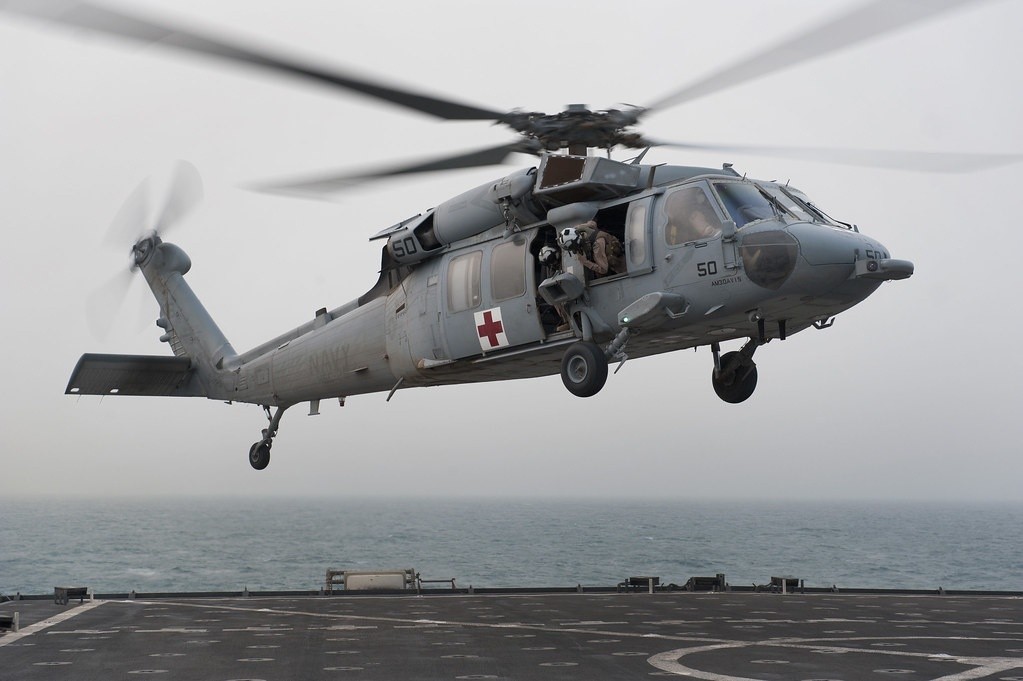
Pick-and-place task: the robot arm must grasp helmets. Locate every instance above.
[539,246,559,268]
[558,228,583,252]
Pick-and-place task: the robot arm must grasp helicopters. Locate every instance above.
[64,0,915,469]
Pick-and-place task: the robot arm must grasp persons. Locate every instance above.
[557,221,626,277]
[690,190,719,236]
[539,245,569,332]
